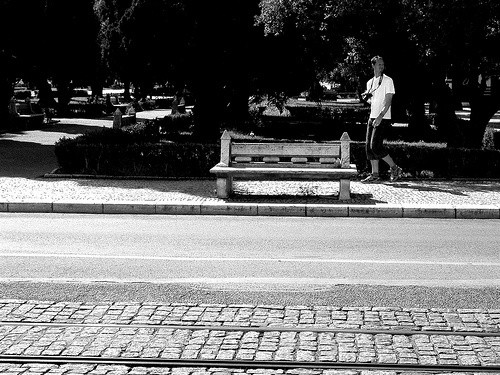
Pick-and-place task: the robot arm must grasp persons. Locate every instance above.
[360,55,403,183]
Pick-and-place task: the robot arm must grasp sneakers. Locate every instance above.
[361,174,380,184]
[387,165,402,182]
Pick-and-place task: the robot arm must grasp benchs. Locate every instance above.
[10,96,45,126]
[106,93,133,116]
[209,129,360,200]
[153,111,193,134]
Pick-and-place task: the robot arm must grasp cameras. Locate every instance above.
[363,93,372,101]
